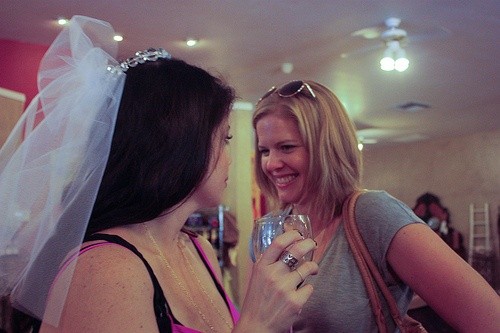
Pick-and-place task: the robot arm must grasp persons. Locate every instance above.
[0,15,319,333]
[248,80,500,333]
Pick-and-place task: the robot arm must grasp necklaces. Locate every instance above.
[143,222,236,333]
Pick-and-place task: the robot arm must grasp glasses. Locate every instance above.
[256,79,317,102]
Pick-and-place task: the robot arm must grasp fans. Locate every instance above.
[346,17,449,53]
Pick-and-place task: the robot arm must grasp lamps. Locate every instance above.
[380,40,409,72]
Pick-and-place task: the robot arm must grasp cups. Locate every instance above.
[253,216,313,315]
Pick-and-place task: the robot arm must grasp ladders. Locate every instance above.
[468,202,492,275]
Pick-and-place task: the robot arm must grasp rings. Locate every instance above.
[295,270,305,281]
[281,251,299,271]
